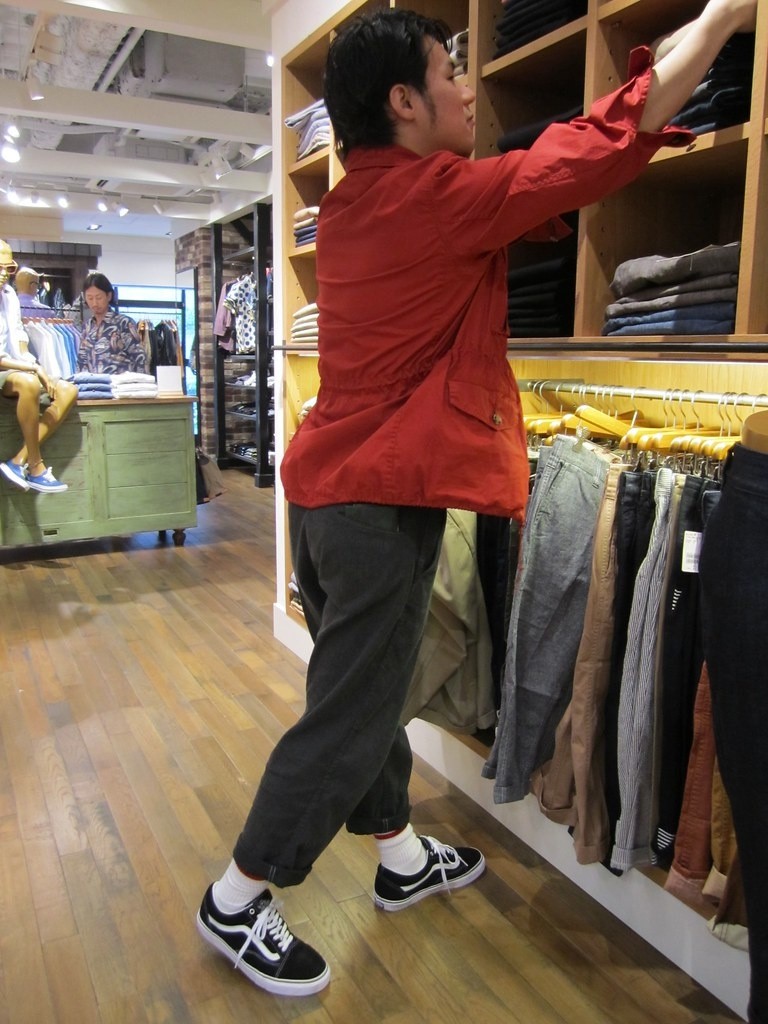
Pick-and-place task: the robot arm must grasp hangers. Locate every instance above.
[21,306,82,325]
[137,311,178,332]
[521,376,768,483]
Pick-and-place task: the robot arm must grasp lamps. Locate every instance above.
[153,197,164,213]
[97,194,109,212]
[31,31,66,66]
[115,196,130,217]
[26,76,45,101]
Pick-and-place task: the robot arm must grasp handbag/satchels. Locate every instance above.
[195,448,230,505]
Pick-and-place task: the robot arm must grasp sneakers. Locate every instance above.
[26,467,68,493]
[371,835,486,912]
[196,880,331,997]
[0,459,30,493]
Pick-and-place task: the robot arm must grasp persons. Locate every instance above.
[0,239,79,492]
[15,266,55,318]
[74,273,147,374]
[195,0,757,997]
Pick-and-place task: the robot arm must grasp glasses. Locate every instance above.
[0,260,19,275]
[30,281,43,289]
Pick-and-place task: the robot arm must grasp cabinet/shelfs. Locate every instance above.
[210,202,274,488]
[0,393,199,561]
[267,0,768,1024]
